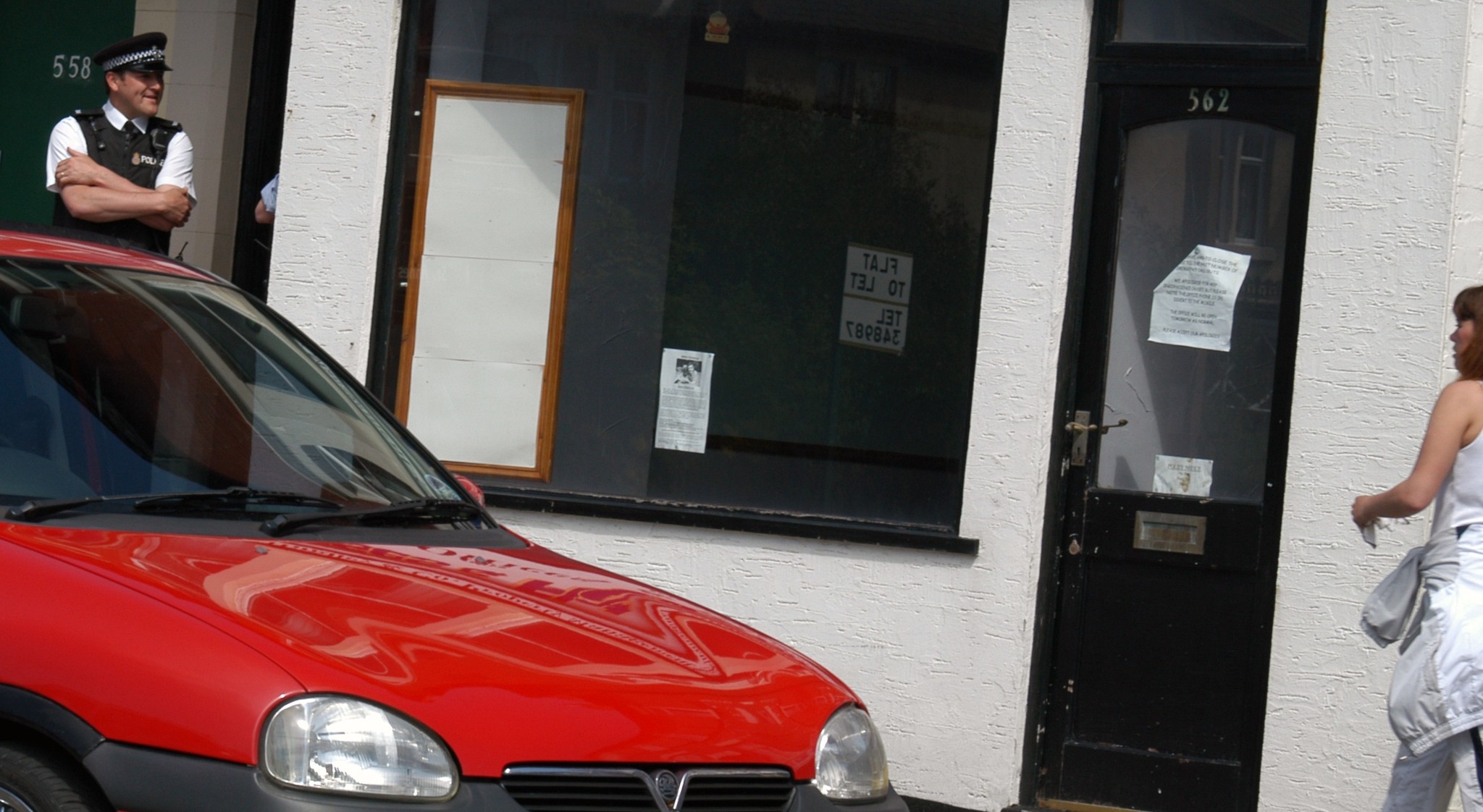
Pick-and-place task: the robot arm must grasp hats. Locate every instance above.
[92,32,173,72]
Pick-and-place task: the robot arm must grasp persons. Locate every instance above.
[46,32,198,256]
[674,362,699,385]
[255,173,279,224]
[1350,286,1483,812]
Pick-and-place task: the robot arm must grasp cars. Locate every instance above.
[0,229,916,811]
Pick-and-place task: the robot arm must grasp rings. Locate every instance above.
[61,172,64,177]
